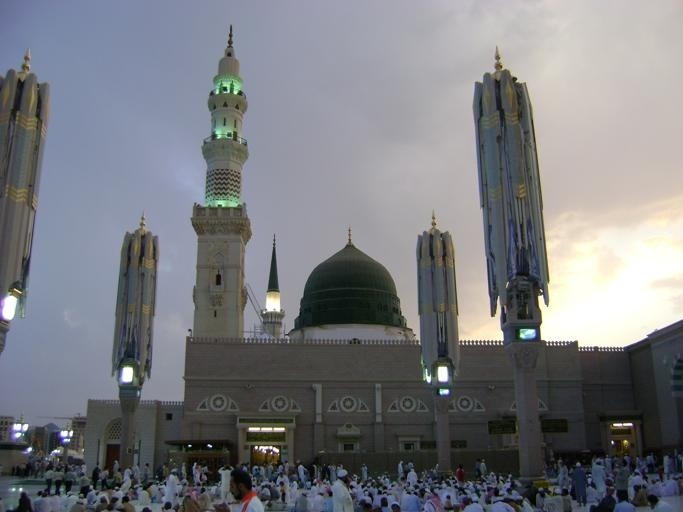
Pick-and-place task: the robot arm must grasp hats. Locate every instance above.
[51,459,683,512]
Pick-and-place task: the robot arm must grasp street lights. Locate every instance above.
[60,422,73,464]
[13,414,28,443]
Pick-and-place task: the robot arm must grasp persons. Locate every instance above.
[6,449,683,512]
